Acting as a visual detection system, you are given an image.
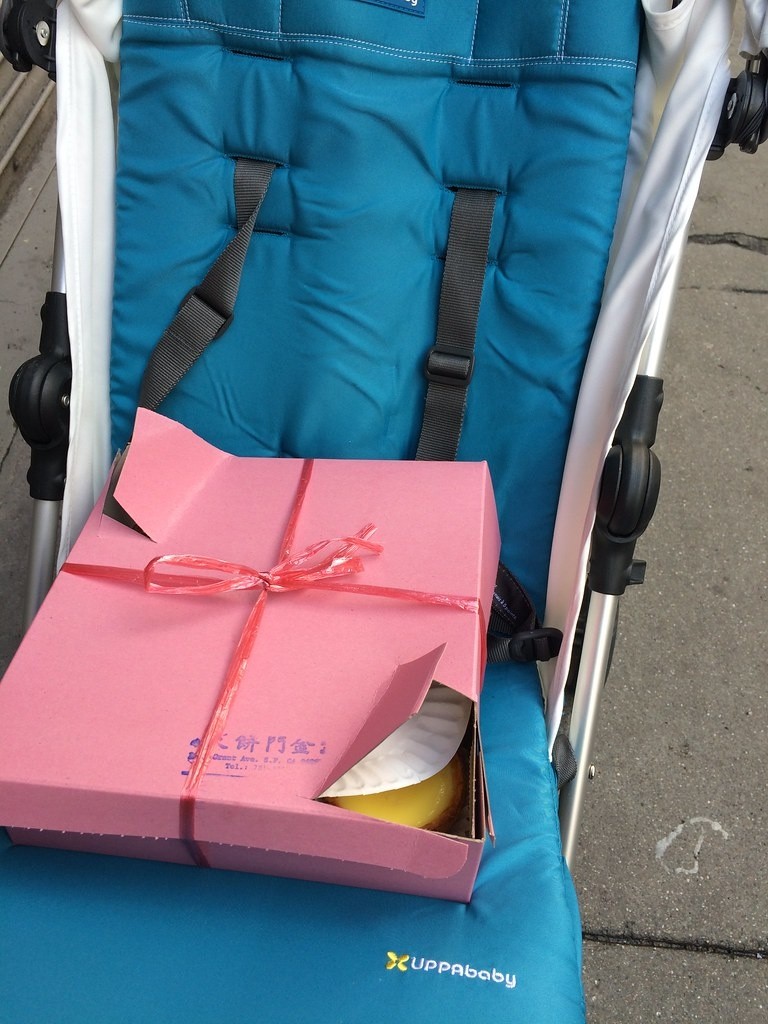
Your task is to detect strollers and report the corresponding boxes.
[1,0,765,1022]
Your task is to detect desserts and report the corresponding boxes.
[330,750,461,832]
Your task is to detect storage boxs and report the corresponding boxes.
[1,407,503,904]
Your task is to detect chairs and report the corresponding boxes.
[0,0,768,1024]
